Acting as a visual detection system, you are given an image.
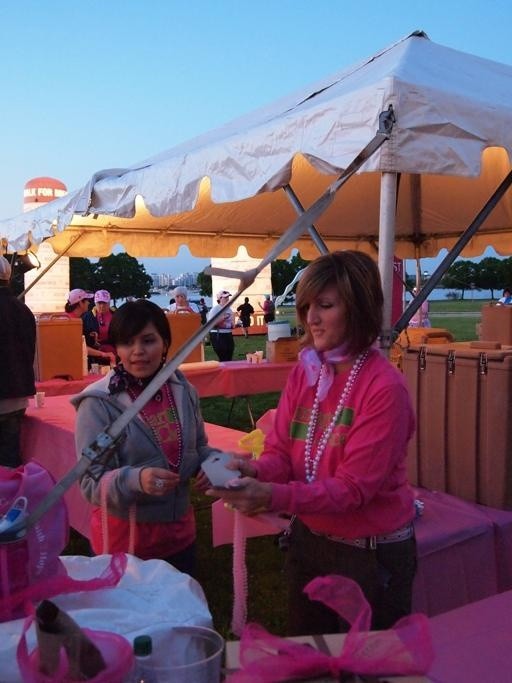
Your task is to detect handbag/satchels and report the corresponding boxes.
[0,458,70,599]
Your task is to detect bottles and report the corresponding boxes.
[237,318,243,327]
[126,634,158,682]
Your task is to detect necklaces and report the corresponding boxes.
[129,381,183,468]
[304,347,370,482]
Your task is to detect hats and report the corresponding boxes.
[94,289,111,302]
[264,294,270,299]
[217,290,232,300]
[68,288,95,306]
[0,256,11,280]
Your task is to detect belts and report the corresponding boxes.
[311,521,414,550]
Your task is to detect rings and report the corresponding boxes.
[239,459,243,471]
[155,479,164,488]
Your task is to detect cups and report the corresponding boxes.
[33,391,45,408]
[89,362,111,375]
[246,349,264,364]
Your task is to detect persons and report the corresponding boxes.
[496,288,512,306]
[0,255,36,470]
[69,299,224,579]
[65,286,275,373]
[206,251,418,636]
[407,287,431,326]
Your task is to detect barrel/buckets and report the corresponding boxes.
[268,321,291,340]
[0,536,33,621]
